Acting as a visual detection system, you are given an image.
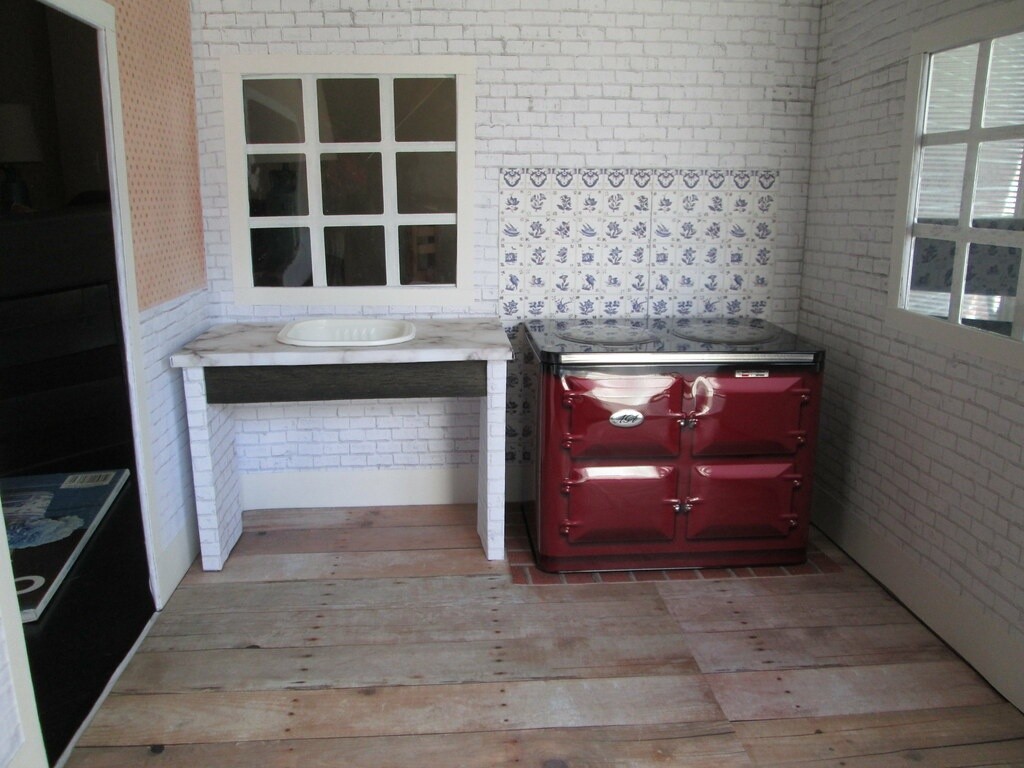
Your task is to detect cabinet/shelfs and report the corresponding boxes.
[531,362,822,574]
